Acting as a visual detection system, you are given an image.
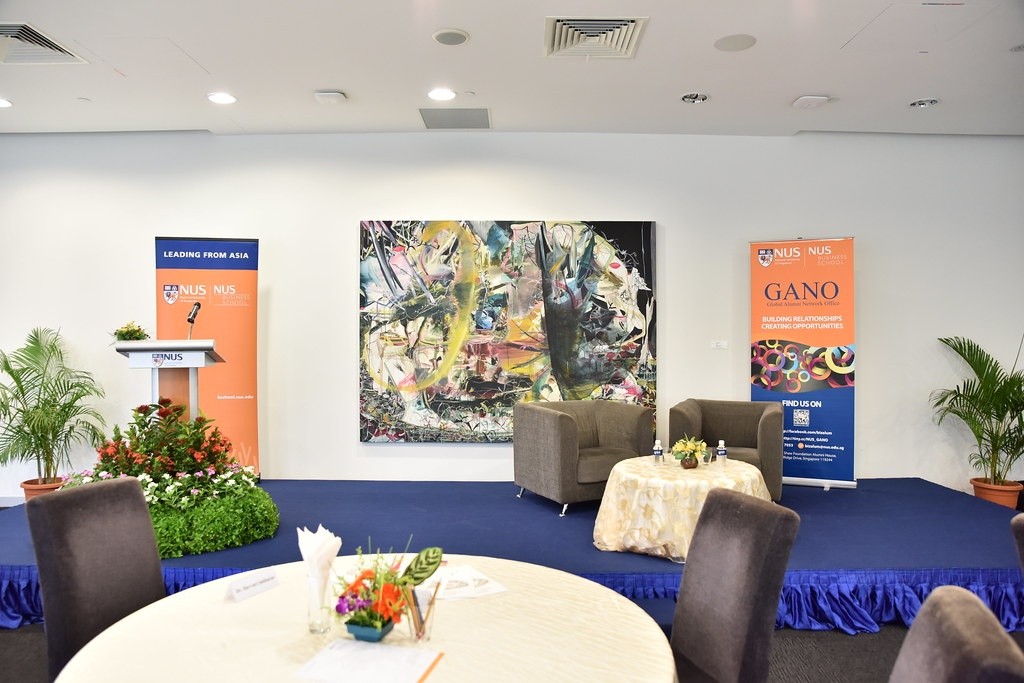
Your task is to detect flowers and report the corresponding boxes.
[671,433,709,458]
[54,399,282,557]
[331,537,448,628]
[114,321,145,335]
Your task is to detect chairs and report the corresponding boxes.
[28,479,166,683]
[886,586,1024,683]
[668,489,801,683]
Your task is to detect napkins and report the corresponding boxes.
[297,525,343,608]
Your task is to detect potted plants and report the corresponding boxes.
[0,327,111,502]
[926,336,1024,509]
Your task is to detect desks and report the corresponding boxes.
[594,456,775,563]
[56,553,681,683]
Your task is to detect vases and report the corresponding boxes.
[121,331,142,341]
[348,616,392,641]
[680,454,699,469]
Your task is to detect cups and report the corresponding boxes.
[662,448,672,462]
[305,598,332,633]
[405,599,434,641]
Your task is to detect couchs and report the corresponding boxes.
[669,397,784,501]
[513,399,653,517]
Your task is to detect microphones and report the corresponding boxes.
[187,302,201,323]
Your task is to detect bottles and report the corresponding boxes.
[653,440,663,466]
[716,440,727,468]
